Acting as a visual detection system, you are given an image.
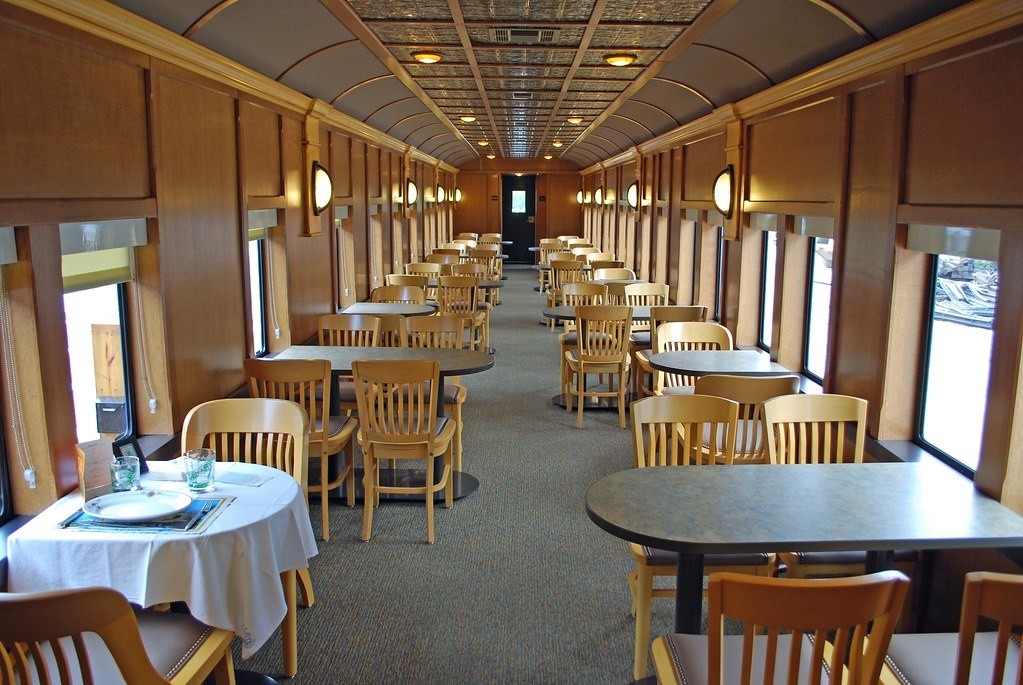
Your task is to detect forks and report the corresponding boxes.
[185,500,213,531]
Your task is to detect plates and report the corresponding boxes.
[83,490,192,522]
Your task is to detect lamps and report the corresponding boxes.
[410,51,445,64]
[627,180,638,211]
[312,160,332,215]
[487,153,496,159]
[544,153,552,160]
[455,187,462,202]
[476,140,489,146]
[407,177,418,207]
[565,116,585,123]
[594,186,603,207]
[553,141,563,147]
[602,53,638,67]
[437,182,445,205]
[458,116,476,123]
[576,190,583,204]
[712,163,734,220]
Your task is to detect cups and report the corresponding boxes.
[109,456,142,493]
[183,448,215,494]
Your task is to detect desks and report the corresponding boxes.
[341,303,435,346]
[542,304,660,409]
[528,246,571,291]
[6,459,319,677]
[421,278,504,354]
[459,253,509,305]
[530,264,591,324]
[649,349,792,419]
[585,461,1023,685]
[272,345,495,502]
[477,240,513,280]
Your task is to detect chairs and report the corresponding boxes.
[0,229,1022,685]
